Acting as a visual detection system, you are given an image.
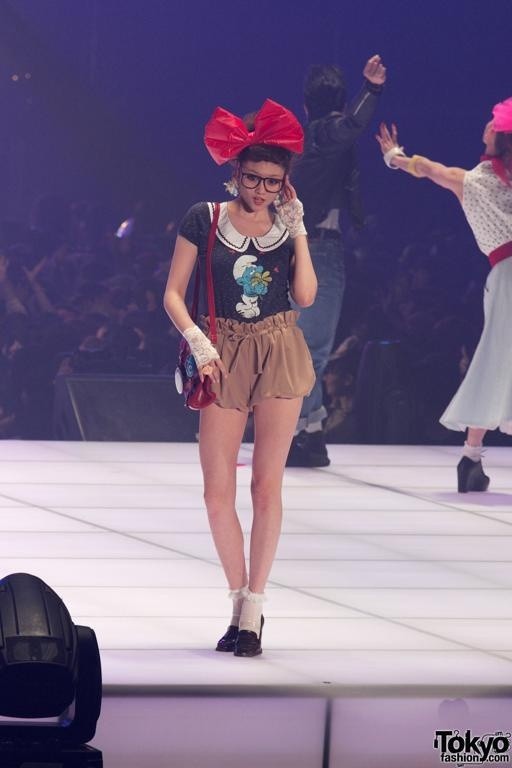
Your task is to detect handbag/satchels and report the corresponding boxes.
[174,336,217,411]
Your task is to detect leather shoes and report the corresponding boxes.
[216,624,239,653]
[234,614,265,657]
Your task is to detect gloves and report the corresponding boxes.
[274,197,309,239]
[182,324,221,368]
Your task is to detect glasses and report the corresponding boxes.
[236,160,285,194]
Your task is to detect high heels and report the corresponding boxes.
[457,455,490,494]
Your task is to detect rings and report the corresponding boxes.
[202,364,213,375]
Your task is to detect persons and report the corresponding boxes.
[162,96,321,657]
[374,93,512,494]
[0,188,493,443]
[284,53,389,468]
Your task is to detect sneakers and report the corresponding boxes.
[284,427,329,468]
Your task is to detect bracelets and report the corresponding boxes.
[383,145,406,170]
